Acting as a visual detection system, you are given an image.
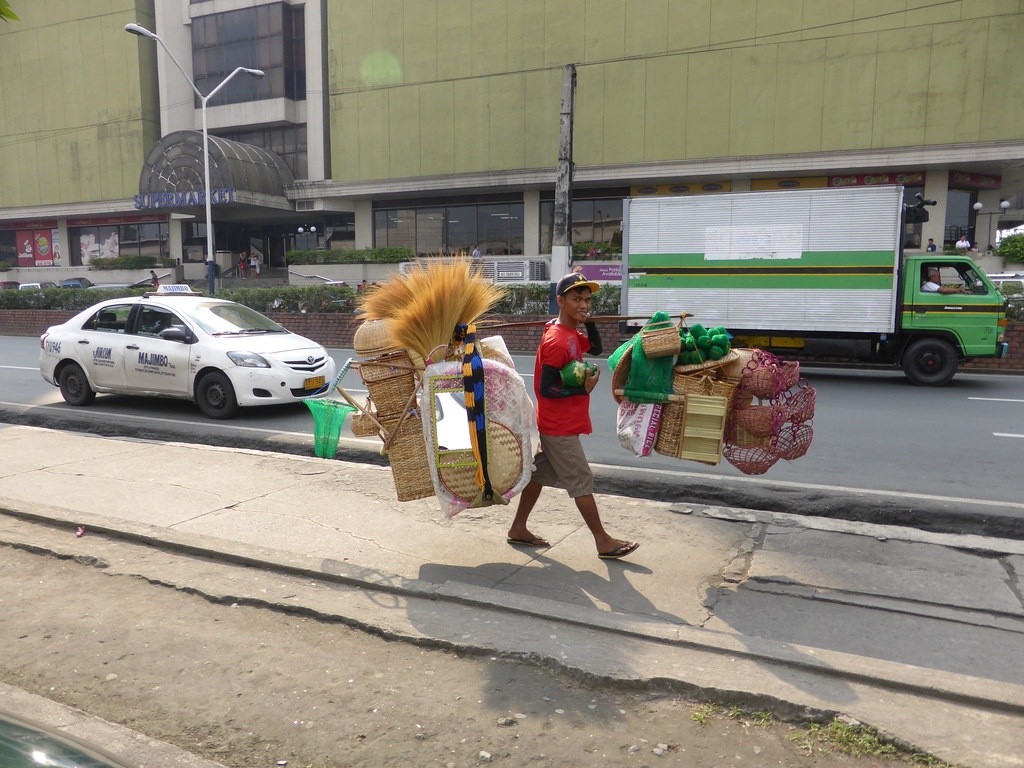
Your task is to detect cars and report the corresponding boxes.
[38,283,336,422]
[61,277,95,290]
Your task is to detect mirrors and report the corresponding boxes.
[429,375,489,468]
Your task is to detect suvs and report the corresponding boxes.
[975,273,1024,311]
[18,281,61,290]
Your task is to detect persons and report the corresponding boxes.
[150,270,159,286]
[926,238,936,252]
[240,250,257,279]
[921,269,964,293]
[505,273,641,558]
[967,242,980,252]
[473,245,480,257]
[956,234,970,248]
[359,279,367,291]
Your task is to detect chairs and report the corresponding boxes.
[97,312,123,328]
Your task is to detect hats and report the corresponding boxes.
[928,269,940,276]
[556,272,600,296]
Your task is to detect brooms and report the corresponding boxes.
[345,247,511,367]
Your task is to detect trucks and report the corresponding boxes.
[616,184,1010,389]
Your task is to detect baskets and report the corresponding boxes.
[351,398,380,437]
[722,348,818,474]
[359,351,436,502]
[652,371,736,466]
[641,321,680,358]
[354,318,405,351]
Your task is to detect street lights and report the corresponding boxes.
[972,201,1011,253]
[123,23,266,299]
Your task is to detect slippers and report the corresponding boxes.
[598,541,639,559]
[507,535,550,547]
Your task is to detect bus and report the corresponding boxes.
[0,282,22,291]
